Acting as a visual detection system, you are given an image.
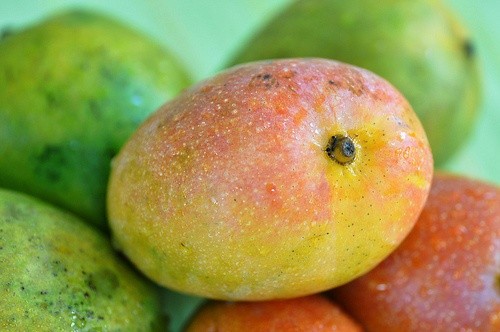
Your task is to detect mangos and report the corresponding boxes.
[0,1,500,332]
